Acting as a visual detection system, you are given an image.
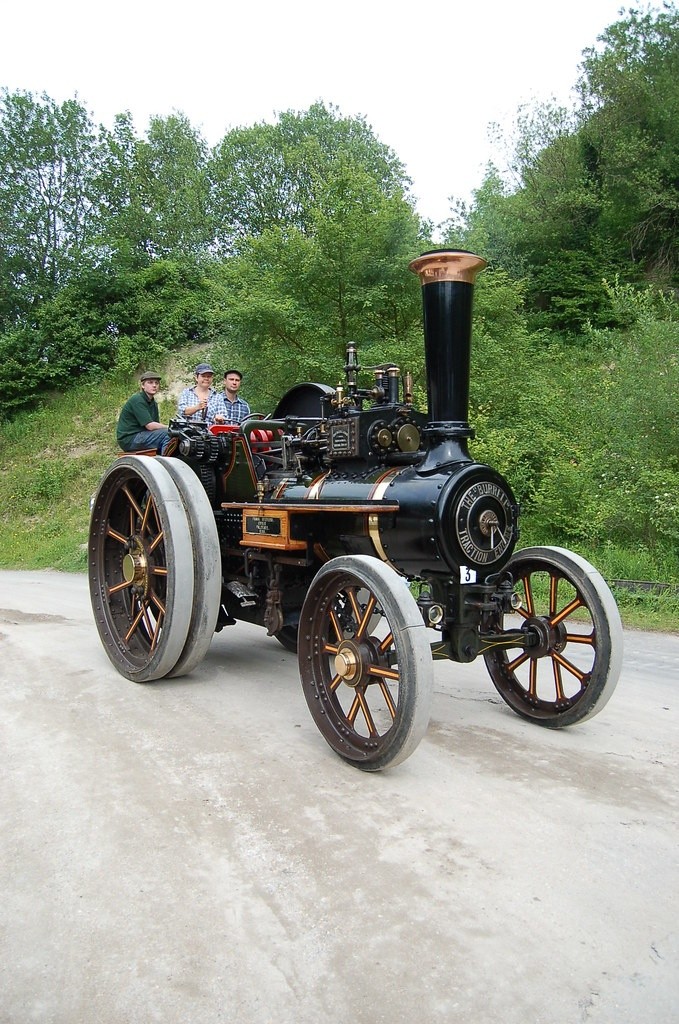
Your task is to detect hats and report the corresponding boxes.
[140,370,162,381]
[195,363,215,374]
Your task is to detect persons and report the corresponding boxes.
[220,369,251,426]
[116,372,172,456]
[177,363,228,428]
